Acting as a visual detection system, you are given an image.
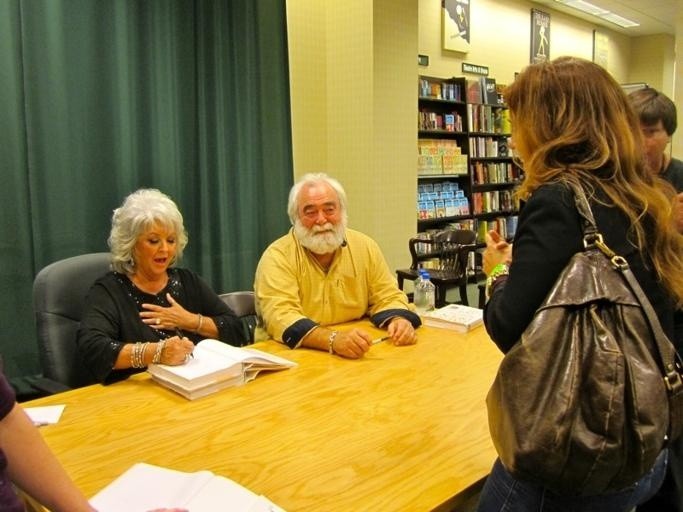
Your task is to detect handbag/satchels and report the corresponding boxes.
[485,235,679,505]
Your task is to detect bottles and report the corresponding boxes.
[411,268,435,312]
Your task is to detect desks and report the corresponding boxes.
[9,301,521,511]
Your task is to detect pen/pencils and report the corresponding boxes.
[372,336,391,344]
[176,329,194,359]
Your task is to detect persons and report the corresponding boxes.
[476,58,683,512]
[628,87,683,233]
[0,363,189,512]
[75,189,246,386]
[253,173,422,360]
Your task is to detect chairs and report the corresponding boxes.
[217,288,267,346]
[29,248,124,396]
[395,226,491,312]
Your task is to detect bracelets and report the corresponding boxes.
[131,339,165,370]
[328,328,337,355]
[486,264,510,287]
[195,315,202,333]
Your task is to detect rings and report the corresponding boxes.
[186,354,190,361]
[156,318,161,324]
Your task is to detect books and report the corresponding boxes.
[417,77,520,276]
[147,338,298,388]
[151,363,289,401]
[422,304,484,333]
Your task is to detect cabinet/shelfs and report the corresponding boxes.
[463,76,524,249]
[415,69,480,292]
